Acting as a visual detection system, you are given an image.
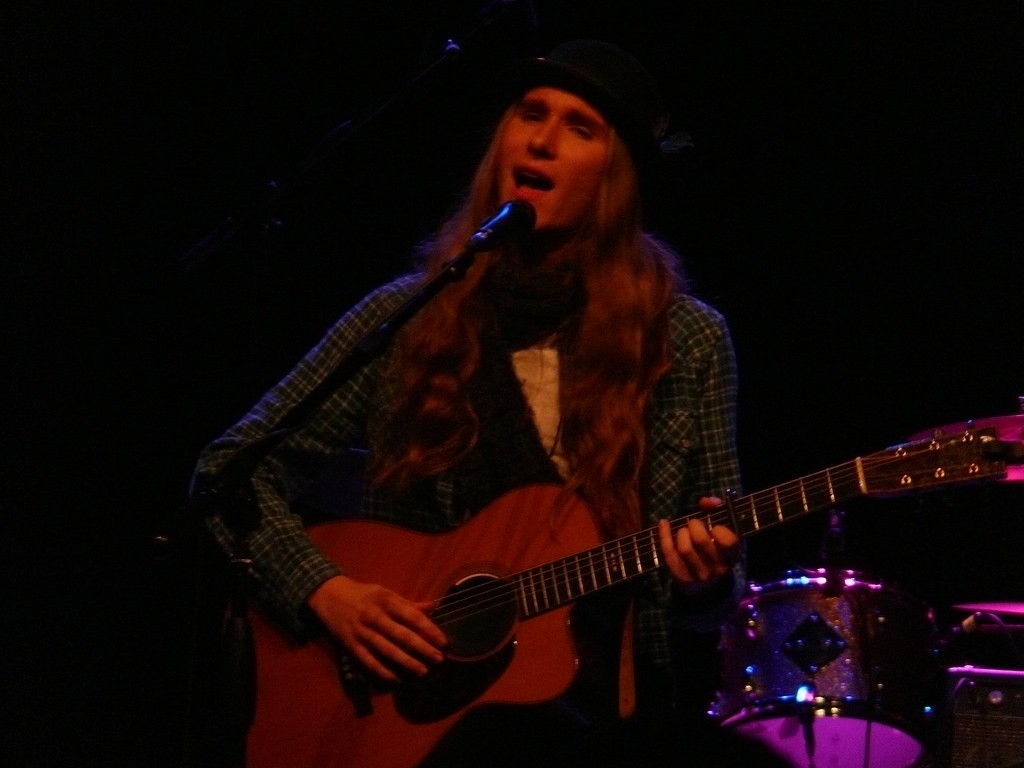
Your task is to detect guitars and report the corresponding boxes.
[247,418,1007,768]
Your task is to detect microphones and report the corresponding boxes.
[469,199,537,252]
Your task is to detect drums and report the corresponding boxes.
[706,565,943,768]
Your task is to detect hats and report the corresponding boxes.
[509,35,669,180]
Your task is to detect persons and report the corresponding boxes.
[187,53,798,768]
